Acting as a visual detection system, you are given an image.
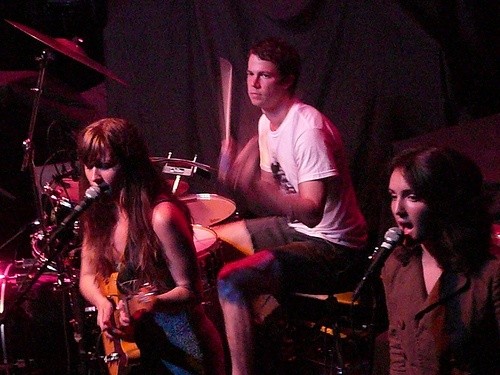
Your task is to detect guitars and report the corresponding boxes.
[97,271,157,375]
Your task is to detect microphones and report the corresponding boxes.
[351,227,405,300]
[54,187,104,236]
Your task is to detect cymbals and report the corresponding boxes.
[2,18,130,90]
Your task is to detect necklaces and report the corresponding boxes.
[119,211,131,222]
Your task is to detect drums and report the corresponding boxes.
[176,194,238,227]
[167,179,189,194]
[191,224,222,302]
[0,258,82,356]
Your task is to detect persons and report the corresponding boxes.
[372,143,500,375]
[205,40,371,375]
[74,117,222,375]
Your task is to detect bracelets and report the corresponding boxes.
[287,196,296,221]
